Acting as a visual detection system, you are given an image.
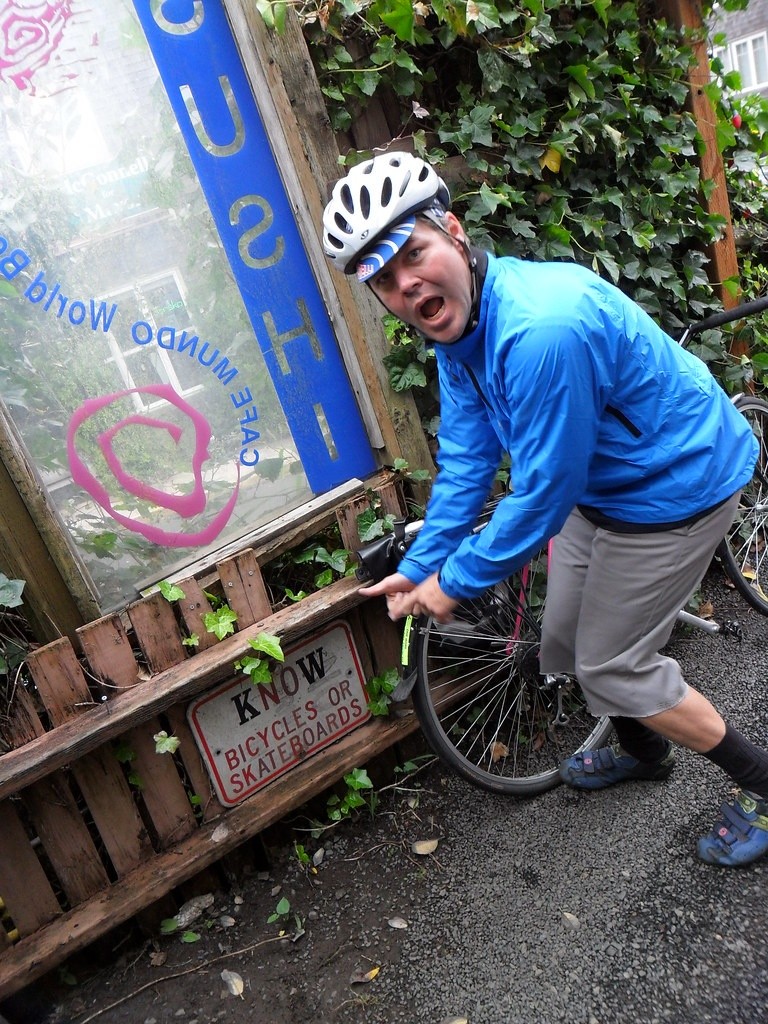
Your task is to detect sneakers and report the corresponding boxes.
[696,789,768,866]
[559,737,675,789]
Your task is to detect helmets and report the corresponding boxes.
[323,151,450,274]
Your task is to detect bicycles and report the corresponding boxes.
[354,299,767,799]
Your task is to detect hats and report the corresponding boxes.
[345,196,445,284]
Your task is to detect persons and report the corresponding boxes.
[320,152,768,868]
